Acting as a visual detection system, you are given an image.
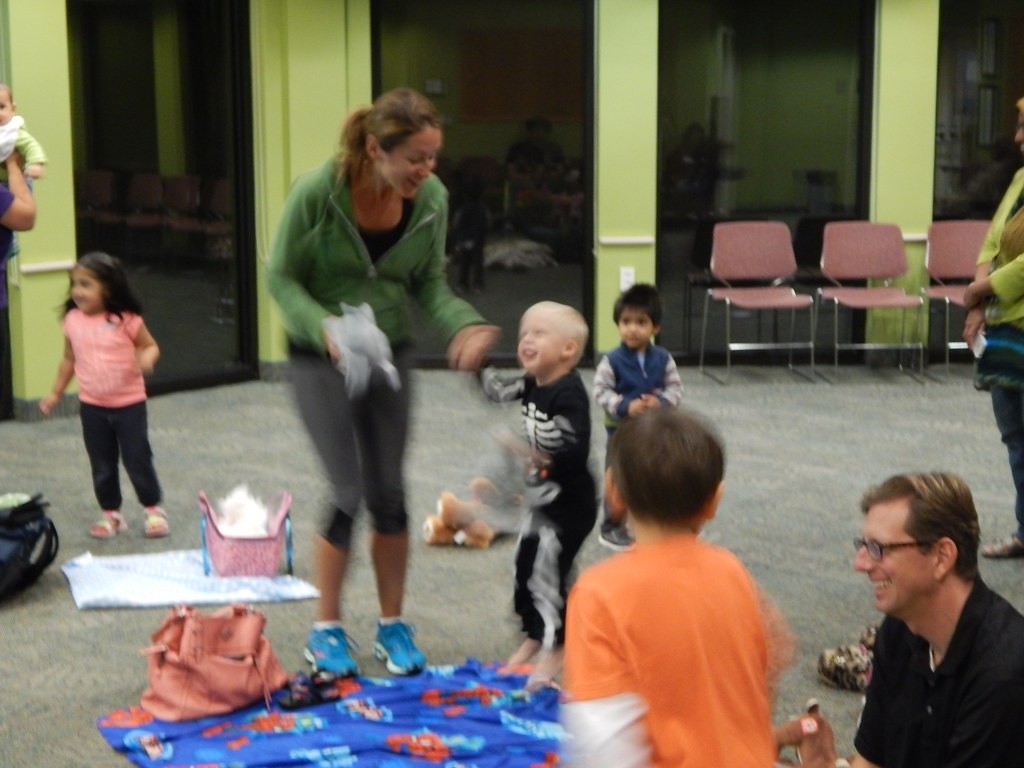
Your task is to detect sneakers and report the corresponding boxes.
[303,627,364,677]
[371,619,426,676]
[598,520,638,552]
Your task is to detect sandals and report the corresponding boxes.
[142,506,169,538]
[89,510,130,539]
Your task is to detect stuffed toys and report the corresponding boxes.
[423,475,526,549]
[817,628,878,696]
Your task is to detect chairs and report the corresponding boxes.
[698,221,819,385]
[677,217,780,354]
[812,222,927,386]
[910,220,992,387]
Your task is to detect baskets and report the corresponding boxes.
[197,488,293,578]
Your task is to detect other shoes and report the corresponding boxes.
[982,535,1024,559]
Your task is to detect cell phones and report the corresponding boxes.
[971,330,987,358]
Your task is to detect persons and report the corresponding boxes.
[842,471,1023,768]
[464,301,599,682]
[263,88,497,678]
[963,101,1024,558]
[36,252,171,539]
[548,406,797,766]
[1,82,46,259]
[591,284,686,552]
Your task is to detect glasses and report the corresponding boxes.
[853,536,932,561]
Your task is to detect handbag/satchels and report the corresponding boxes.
[139,603,289,722]
[0,493,60,603]
